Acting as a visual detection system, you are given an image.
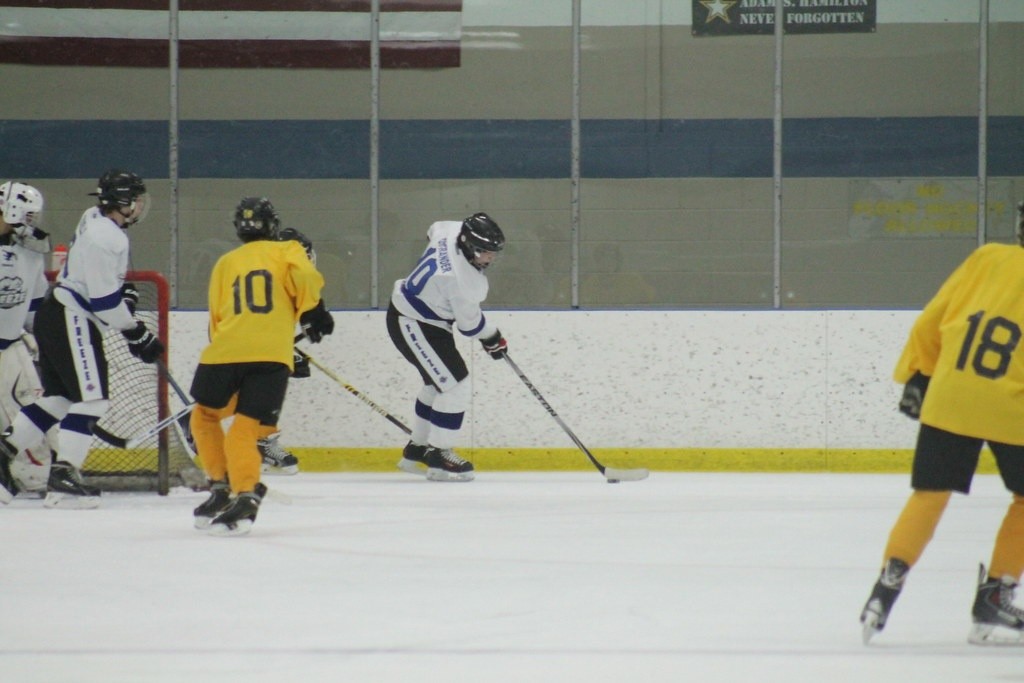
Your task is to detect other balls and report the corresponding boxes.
[607,478,620,483]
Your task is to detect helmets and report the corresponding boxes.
[457,212,506,272]
[279,227,316,269]
[0,181,43,228]
[1016,201,1024,247]
[87,169,152,230]
[233,195,281,244]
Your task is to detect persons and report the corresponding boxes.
[0,168,334,537]
[857,201,1024,647]
[386,211,509,483]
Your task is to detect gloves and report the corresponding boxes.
[120,319,165,364]
[122,282,140,317]
[898,370,931,419]
[290,347,311,379]
[299,298,336,345]
[478,327,508,359]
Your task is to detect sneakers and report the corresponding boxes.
[0,436,102,510]
[193,479,268,537]
[256,429,299,476]
[967,562,1024,645]
[860,558,910,644]
[174,408,203,470]
[396,440,474,481]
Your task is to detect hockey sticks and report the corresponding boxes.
[90,325,316,452]
[155,361,190,404]
[293,347,412,435]
[500,350,650,481]
